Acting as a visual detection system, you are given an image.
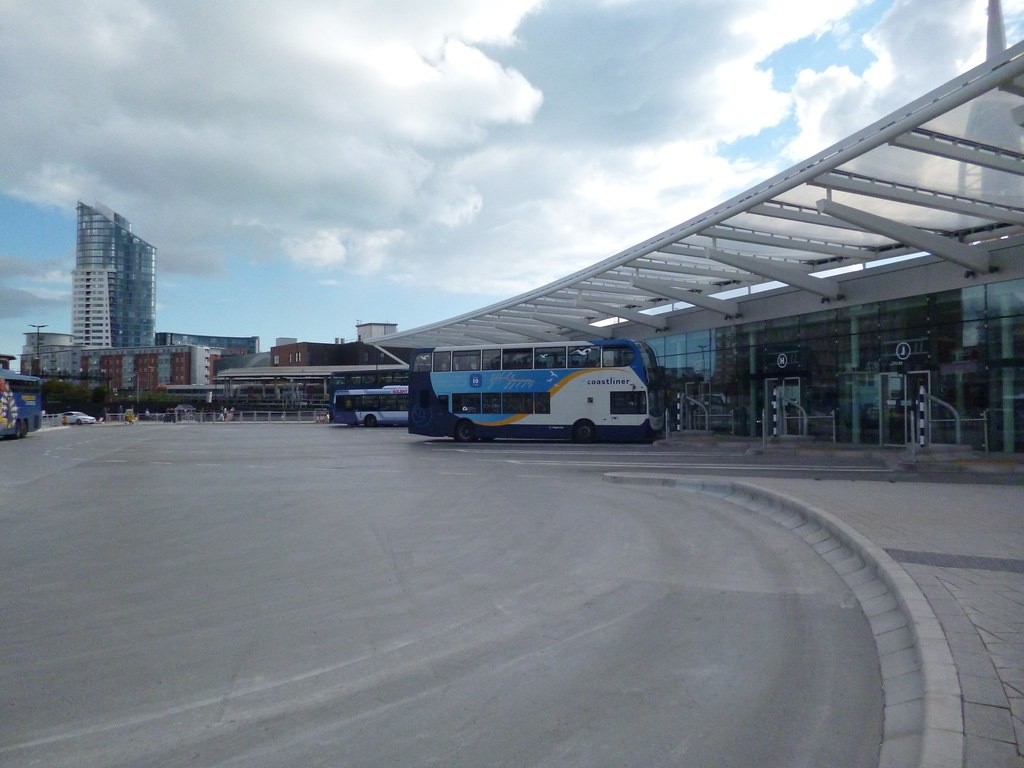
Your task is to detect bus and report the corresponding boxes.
[327,369,408,423]
[333,385,409,428]
[407,339,666,445]
[0,368,42,439]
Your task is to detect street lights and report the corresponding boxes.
[29,325,47,358]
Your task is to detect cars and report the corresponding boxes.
[174,404,196,412]
[62,411,96,425]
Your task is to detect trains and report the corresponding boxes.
[155,383,330,409]
